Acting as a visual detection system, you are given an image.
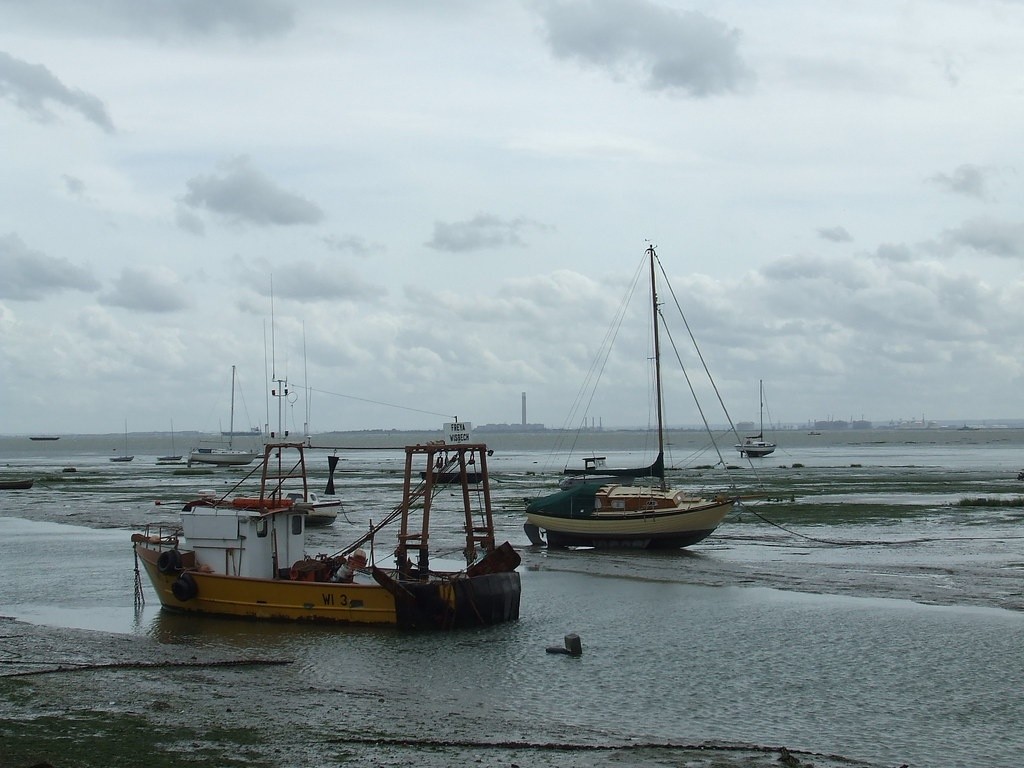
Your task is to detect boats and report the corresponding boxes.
[0,480,33,488]
[133,379,520,625]
[815,414,1007,429]
[29,436,60,440]
[250,490,341,524]
[806,431,821,435]
[63,467,76,472]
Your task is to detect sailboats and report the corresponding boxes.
[188,365,258,470]
[523,245,741,550]
[157,417,183,460]
[734,379,778,457]
[109,419,134,462]
